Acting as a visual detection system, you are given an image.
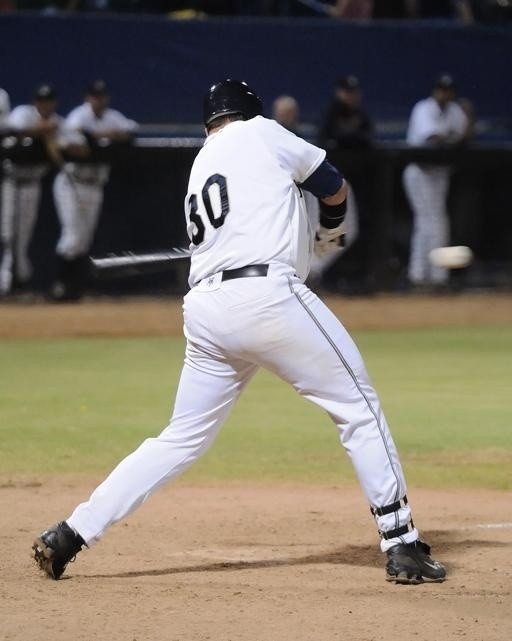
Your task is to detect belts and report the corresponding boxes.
[195,264,269,285]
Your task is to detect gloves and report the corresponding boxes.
[316,224,344,259]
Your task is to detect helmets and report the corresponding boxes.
[203,77,263,128]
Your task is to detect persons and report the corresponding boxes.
[29,75,448,585]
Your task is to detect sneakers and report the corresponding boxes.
[29,520,82,580]
[386,539,445,582]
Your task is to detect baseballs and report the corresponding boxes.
[430,245,474,268]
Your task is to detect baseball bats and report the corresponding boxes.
[90,233,347,270]
